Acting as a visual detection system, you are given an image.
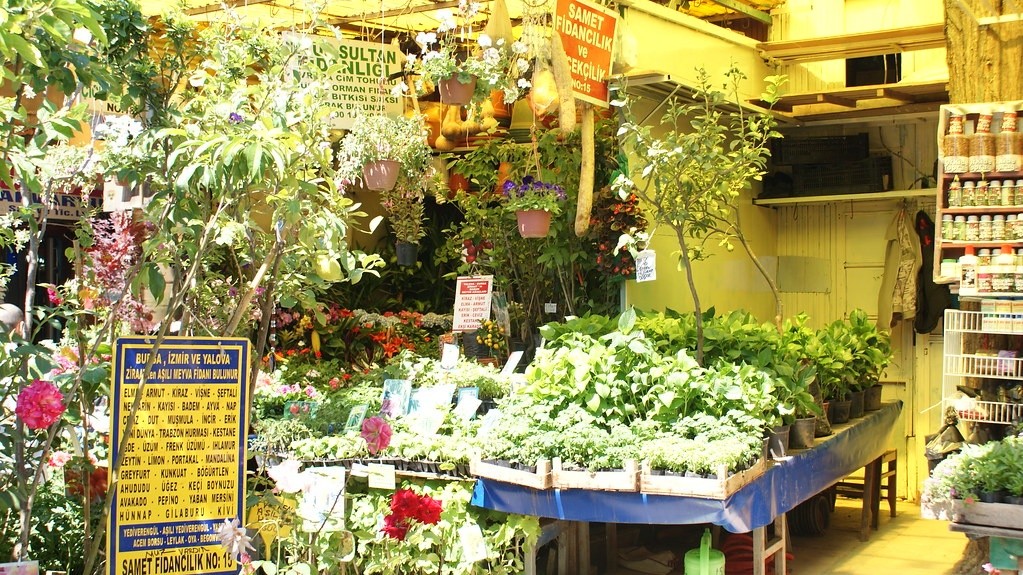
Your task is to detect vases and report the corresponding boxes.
[514,209,552,238]
[979,433,1023,505]
[362,157,402,192]
[436,72,479,106]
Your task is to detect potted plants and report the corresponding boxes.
[468,304,893,501]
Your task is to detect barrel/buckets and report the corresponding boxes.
[683,528,725,575]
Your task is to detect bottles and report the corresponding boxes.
[995,245,1014,265]
[960,244,977,296]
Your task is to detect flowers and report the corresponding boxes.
[500,174,565,214]
[335,112,438,246]
[2,0,707,575]
[924,447,978,506]
[388,0,533,107]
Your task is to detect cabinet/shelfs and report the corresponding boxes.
[932,101,1023,431]
[583,0,948,132]
